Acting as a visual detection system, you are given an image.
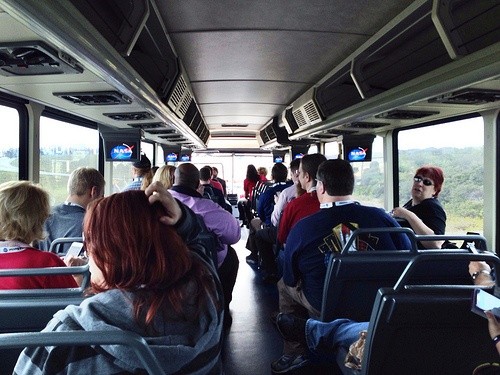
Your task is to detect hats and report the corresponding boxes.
[132,154,151,169]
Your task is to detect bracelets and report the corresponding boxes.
[493,335,500,345]
[472,269,490,280]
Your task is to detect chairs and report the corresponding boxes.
[252,180,500,375]
[0,236,168,375]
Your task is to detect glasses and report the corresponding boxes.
[296,170,304,174]
[315,179,321,184]
[414,175,434,187]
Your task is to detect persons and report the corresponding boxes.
[121,155,241,310]
[0,180,85,290]
[236,154,500,375]
[36,168,106,254]
[12,182,224,375]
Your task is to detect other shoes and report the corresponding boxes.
[222,313,232,328]
[246,254,258,260]
[241,222,247,227]
[253,272,270,282]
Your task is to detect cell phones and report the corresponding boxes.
[470,286,500,319]
[64,242,84,267]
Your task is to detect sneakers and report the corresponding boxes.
[270,351,309,373]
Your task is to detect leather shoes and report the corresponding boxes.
[273,312,307,343]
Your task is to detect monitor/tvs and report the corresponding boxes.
[344,141,372,162]
[164,149,179,162]
[273,155,284,162]
[292,151,307,159]
[180,153,191,161]
[104,140,138,162]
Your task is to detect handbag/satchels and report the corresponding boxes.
[344,332,367,370]
